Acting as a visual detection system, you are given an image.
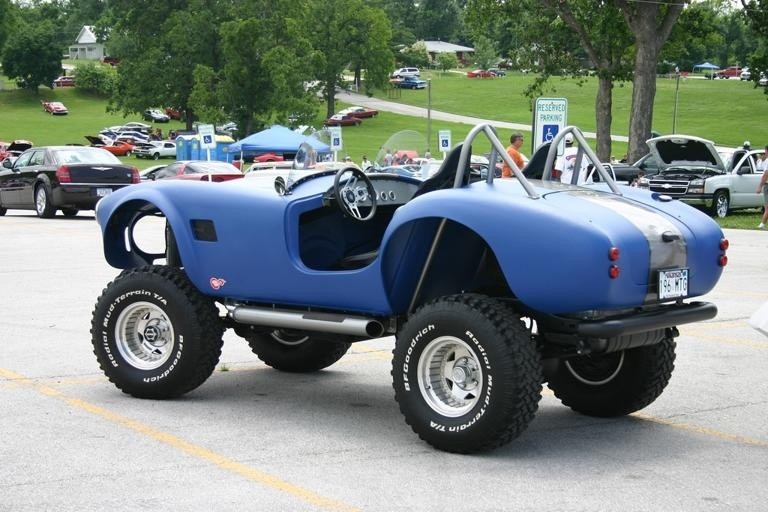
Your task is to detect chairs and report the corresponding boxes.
[510,141,556,181]
[339,145,472,270]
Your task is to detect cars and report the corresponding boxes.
[467,68,505,78]
[54,75,75,87]
[42,101,69,115]
[323,107,378,127]
[741,66,768,86]
[143,106,186,123]
[0,141,245,218]
[16,78,39,88]
[389,66,427,89]
[85,122,196,160]
[216,119,239,135]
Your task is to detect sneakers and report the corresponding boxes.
[757,222,765,229]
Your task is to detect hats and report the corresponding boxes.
[564,132,573,143]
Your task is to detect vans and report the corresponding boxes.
[100,56,118,66]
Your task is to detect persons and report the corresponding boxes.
[621,154,627,163]
[342,145,431,172]
[631,171,651,191]
[554,133,589,186]
[755,153,767,170]
[755,144,767,228]
[146,127,177,142]
[740,140,757,173]
[501,131,525,178]
[610,155,618,163]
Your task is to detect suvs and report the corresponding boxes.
[718,66,742,79]
